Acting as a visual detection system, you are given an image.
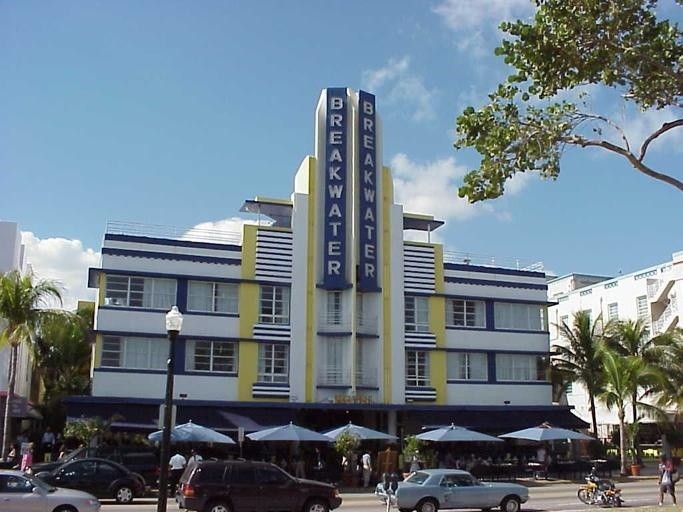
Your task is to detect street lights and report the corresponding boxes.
[154,303,184,511]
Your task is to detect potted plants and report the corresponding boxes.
[332,425,361,488]
[621,419,641,476]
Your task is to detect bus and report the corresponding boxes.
[606,424,681,459]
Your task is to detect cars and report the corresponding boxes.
[0,468,101,511]
[374,467,529,511]
[31,457,147,504]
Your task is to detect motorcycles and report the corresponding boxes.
[576,460,624,507]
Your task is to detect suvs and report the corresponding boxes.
[174,457,341,511]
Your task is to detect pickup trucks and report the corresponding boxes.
[23,442,158,486]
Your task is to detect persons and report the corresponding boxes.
[442,449,489,470]
[657,454,676,507]
[536,443,548,464]
[360,448,372,489]
[6,425,61,462]
[168,449,187,498]
[187,447,203,467]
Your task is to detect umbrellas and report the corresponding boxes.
[403,422,506,454]
[148,419,237,456]
[496,420,596,480]
[245,421,332,472]
[321,420,401,443]
